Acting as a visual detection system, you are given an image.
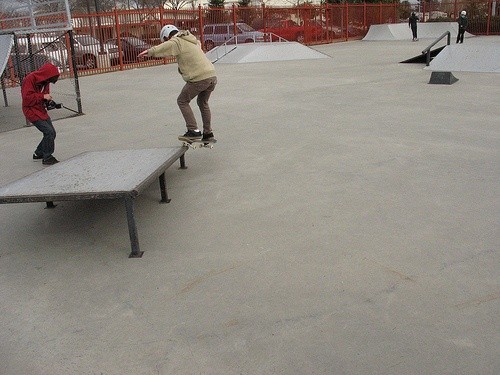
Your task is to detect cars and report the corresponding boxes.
[103,36,158,64]
[309,18,345,40]
[347,20,368,34]
[55,34,126,70]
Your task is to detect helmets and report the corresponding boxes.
[160,25,179,43]
[461,11,466,15]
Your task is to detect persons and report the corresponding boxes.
[137,25,217,142]
[21,62,59,165]
[456,10,468,44]
[409,12,419,41]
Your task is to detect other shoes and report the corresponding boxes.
[33,153,44,162]
[179,130,202,138]
[42,155,60,167]
[202,132,215,146]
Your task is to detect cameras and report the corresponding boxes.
[44,99,63,110]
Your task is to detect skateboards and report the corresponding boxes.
[178,137,218,150]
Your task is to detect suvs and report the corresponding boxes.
[257,17,326,43]
[9,35,69,74]
[200,22,272,51]
